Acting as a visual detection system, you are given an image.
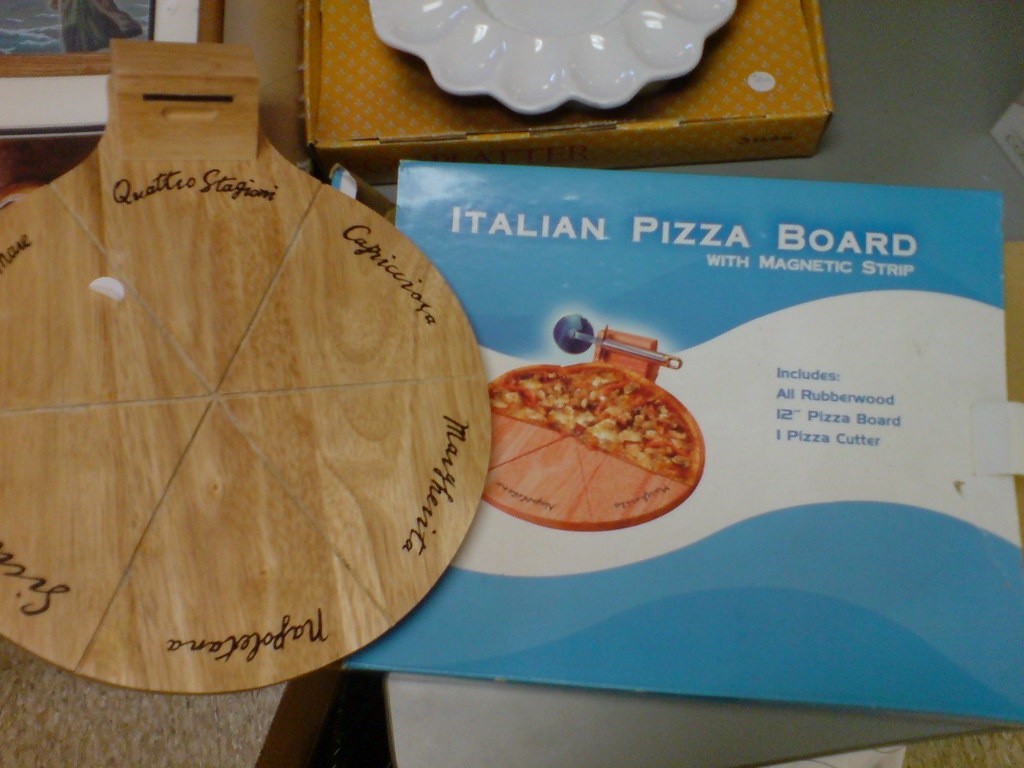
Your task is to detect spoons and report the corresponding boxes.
[554,313,683,371]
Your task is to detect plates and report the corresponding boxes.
[370,1,738,116]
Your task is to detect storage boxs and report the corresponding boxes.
[305,0,835,188]
[328,157,1023,724]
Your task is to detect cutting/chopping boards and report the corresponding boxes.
[481,327,706,532]
[1,38,492,695]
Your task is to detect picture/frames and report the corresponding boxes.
[0,1,225,138]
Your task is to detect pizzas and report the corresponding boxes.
[487,365,702,488]
